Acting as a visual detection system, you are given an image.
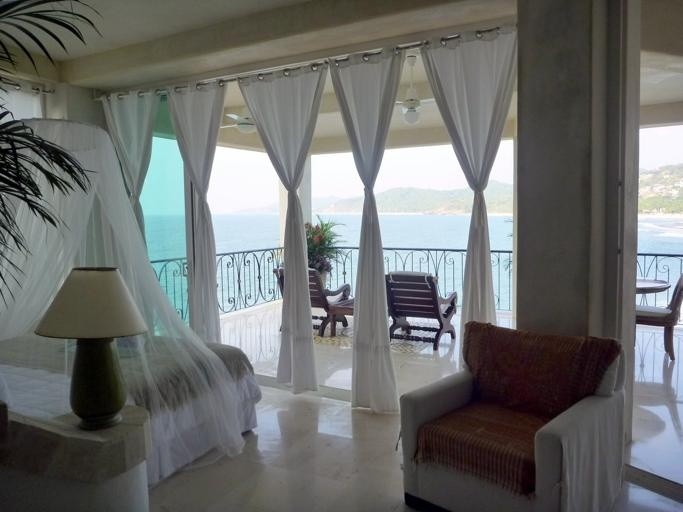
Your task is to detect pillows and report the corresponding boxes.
[464,322,621,413]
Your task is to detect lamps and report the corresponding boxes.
[34,267,148,428]
[405,109,419,124]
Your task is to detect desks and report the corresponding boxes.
[635,280,669,293]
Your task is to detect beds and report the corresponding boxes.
[0,335,262,489]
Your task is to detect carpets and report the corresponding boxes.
[312,321,434,355]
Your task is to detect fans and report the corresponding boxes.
[396,56,435,109]
[220,113,258,135]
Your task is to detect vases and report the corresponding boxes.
[315,271,326,287]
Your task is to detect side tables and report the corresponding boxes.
[327,300,354,336]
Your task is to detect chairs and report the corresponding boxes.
[273,268,349,337]
[636,275,682,361]
[385,273,457,349]
[401,321,625,512]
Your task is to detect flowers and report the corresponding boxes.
[304,213,348,273]
